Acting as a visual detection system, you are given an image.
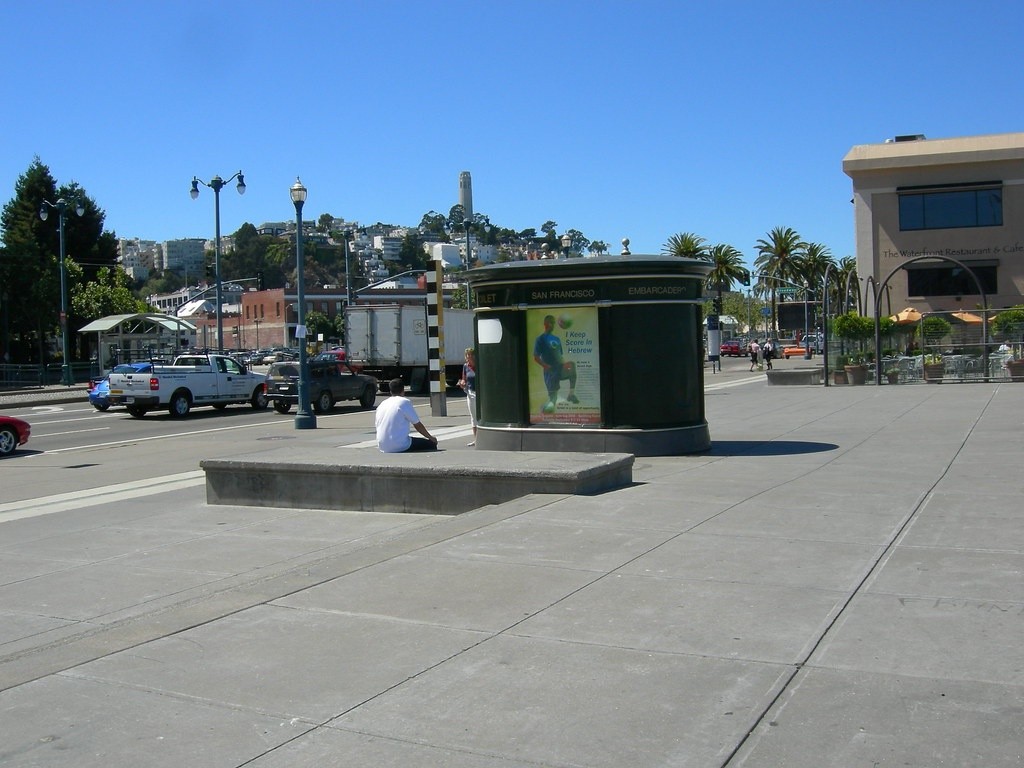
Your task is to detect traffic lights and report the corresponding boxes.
[743,272,750,286]
[712,296,723,313]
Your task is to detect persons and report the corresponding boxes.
[748,339,761,372]
[906,342,920,356]
[999,341,1010,367]
[460,349,476,446]
[764,339,773,370]
[375,379,438,452]
[534,317,579,413]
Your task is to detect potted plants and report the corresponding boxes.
[988,304,1024,382]
[833,349,848,385]
[885,363,900,383]
[917,316,951,384]
[833,310,869,385]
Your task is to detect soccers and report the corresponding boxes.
[540,402,555,415]
[558,313,574,330]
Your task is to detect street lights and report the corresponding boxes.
[803,280,811,360]
[188,170,246,354]
[562,235,572,259]
[289,175,317,429]
[39,195,85,386]
[449,217,491,311]
[251,320,263,350]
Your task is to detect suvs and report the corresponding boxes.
[263,362,379,415]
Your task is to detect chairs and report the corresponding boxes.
[192,359,203,365]
[865,354,1010,384]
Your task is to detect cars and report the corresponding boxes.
[758,340,782,359]
[146,346,363,374]
[720,340,748,357]
[0,415,31,457]
[794,331,824,354]
[89,362,161,412]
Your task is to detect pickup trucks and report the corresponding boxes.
[105,355,269,420]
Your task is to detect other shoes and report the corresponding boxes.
[567,395,580,404]
[468,441,475,446]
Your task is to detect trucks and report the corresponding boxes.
[345,303,477,394]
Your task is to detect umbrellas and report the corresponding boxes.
[887,307,924,329]
[988,314,996,323]
[952,308,982,346]
[918,309,968,353]
